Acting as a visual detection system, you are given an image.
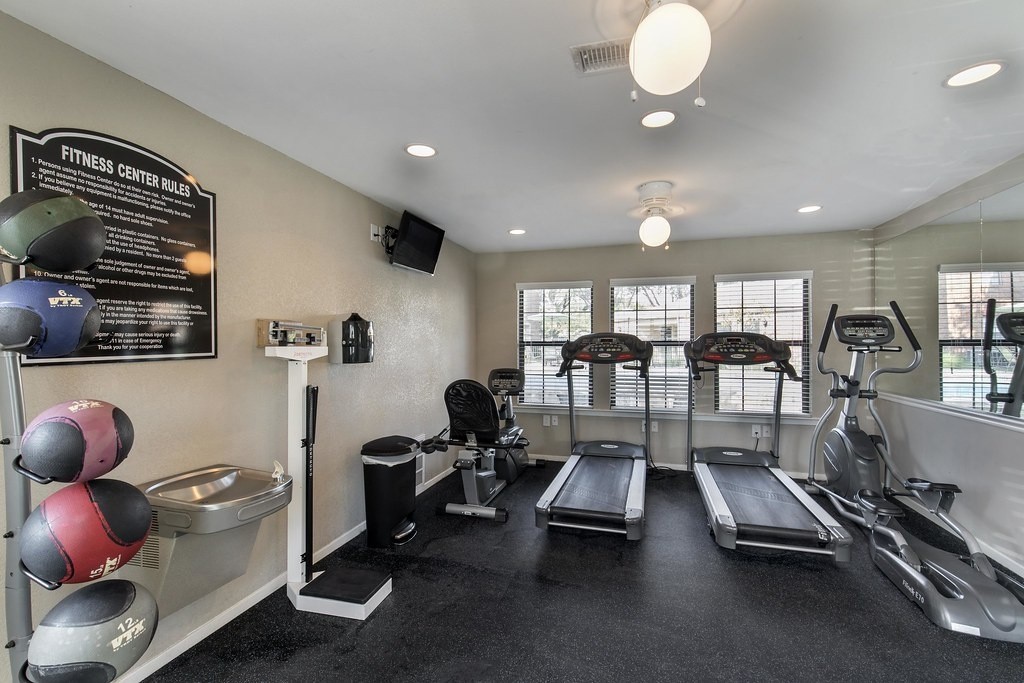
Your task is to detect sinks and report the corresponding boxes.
[114,463,294,621]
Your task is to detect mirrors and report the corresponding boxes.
[875,181,1024,422]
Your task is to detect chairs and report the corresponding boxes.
[445,379,510,443]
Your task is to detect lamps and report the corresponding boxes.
[628,1,711,108]
[638,182,672,251]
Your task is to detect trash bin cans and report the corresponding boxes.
[360,435,422,547]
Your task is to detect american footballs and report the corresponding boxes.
[19,478,153,583]
[0,189,107,273]
[0,276,102,356]
[20,398,134,482]
[27,578,160,683]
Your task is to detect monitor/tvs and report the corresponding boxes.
[390,210,445,276]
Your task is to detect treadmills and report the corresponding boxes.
[534,332,653,540]
[683,331,855,565]
